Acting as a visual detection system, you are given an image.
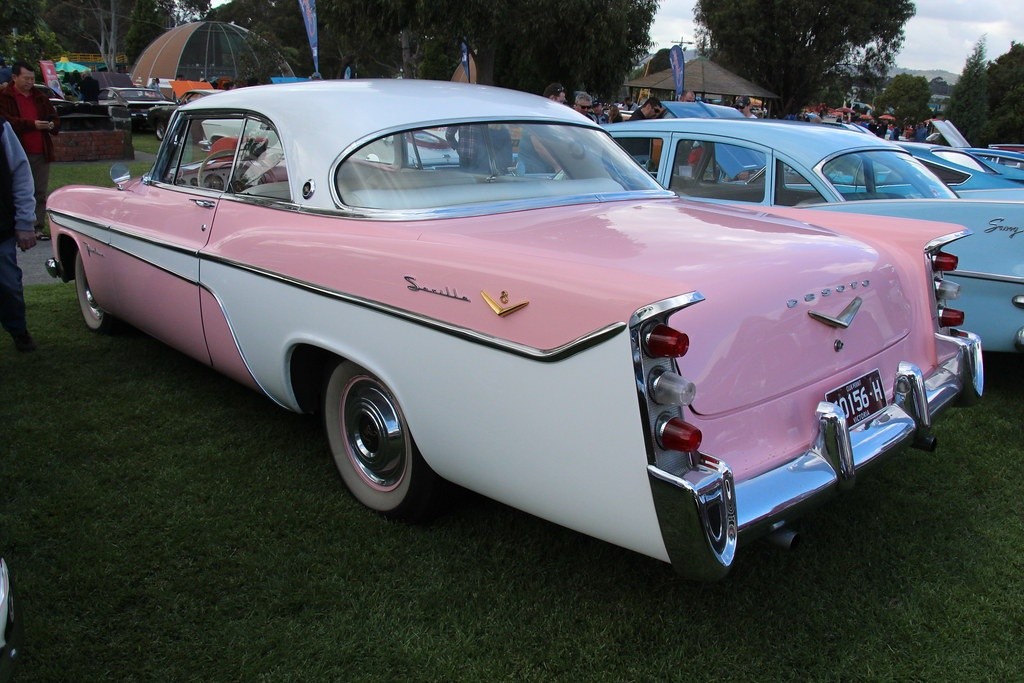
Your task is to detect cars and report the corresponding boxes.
[511,101,1024,352]
[33,71,463,170]
[44,79,984,576]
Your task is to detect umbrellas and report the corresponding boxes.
[834,108,855,113]
[879,115,895,119]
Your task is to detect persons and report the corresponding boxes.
[628,97,662,164]
[869,120,888,139]
[623,96,639,111]
[687,141,702,177]
[889,123,899,141]
[0,114,37,352]
[224,82,236,90]
[445,120,512,173]
[516,83,566,174]
[915,122,927,142]
[0,56,60,241]
[573,92,623,125]
[148,77,160,90]
[733,95,757,118]
[836,116,842,122]
[62,70,100,102]
[680,90,695,102]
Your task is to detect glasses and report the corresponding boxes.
[552,87,565,95]
[653,107,660,114]
[578,104,593,111]
[735,106,745,109]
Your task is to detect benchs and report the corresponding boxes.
[336,161,615,210]
[685,180,790,205]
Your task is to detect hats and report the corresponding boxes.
[733,95,750,106]
[592,100,602,106]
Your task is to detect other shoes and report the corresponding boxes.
[10,326,37,352]
[34,224,52,241]
